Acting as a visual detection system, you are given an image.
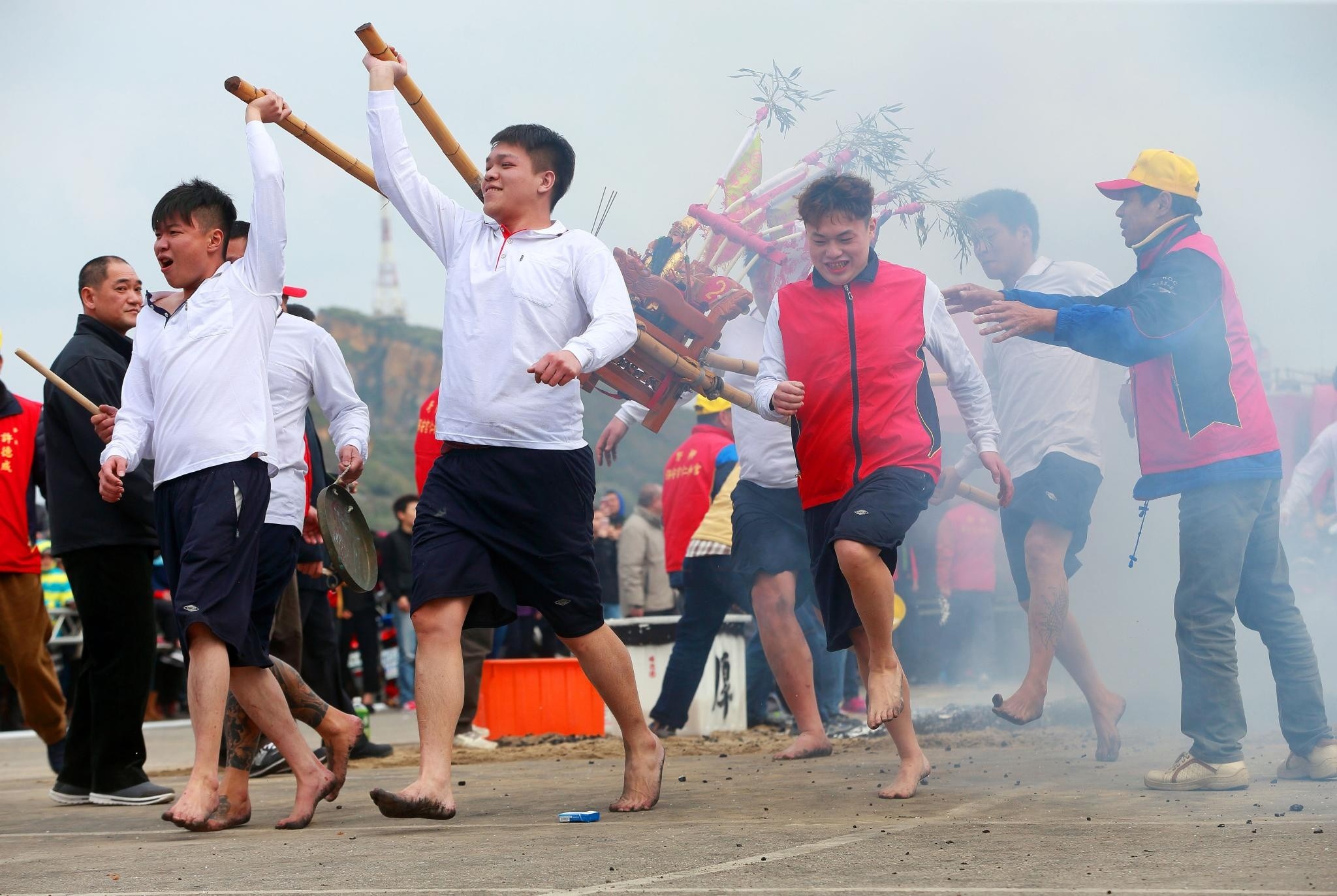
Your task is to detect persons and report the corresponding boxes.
[927,189,1130,761]
[936,147,1337,789]
[746,171,1014,799]
[362,43,666,820]
[0,89,572,833]
[590,383,1019,738]
[1258,360,1337,732]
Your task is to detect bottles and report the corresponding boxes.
[352,697,370,741]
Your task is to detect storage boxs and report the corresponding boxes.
[561,609,750,740]
[474,659,608,741]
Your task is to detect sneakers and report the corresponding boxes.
[89,778,175,806]
[1276,737,1337,779]
[50,780,92,805]
[247,743,287,779]
[1144,752,1249,792]
[315,745,328,763]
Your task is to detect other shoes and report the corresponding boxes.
[453,724,498,750]
[347,733,392,759]
[48,702,74,773]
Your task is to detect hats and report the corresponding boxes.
[694,391,732,416]
[1094,149,1202,203]
